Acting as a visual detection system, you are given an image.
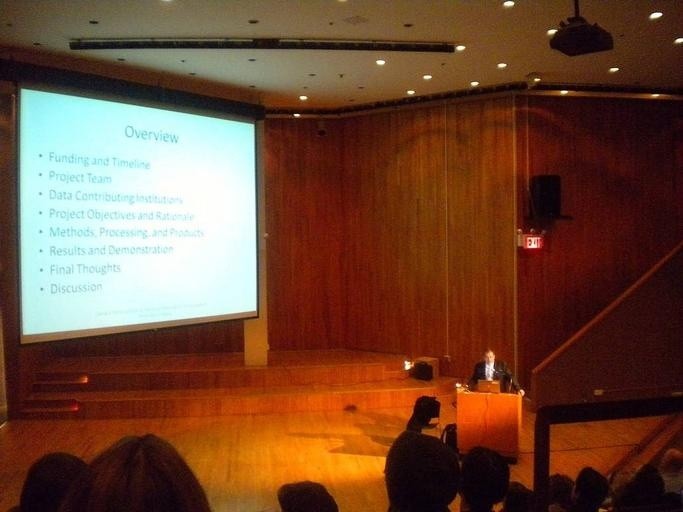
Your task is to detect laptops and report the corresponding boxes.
[478,380,500,393]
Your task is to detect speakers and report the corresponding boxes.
[531,176,562,217]
[415,364,432,380]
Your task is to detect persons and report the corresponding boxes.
[466,348,525,396]
[21,432,210,511]
[277,480,339,512]
[385,398,683,512]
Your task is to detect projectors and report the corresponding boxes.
[550,24,613,57]
